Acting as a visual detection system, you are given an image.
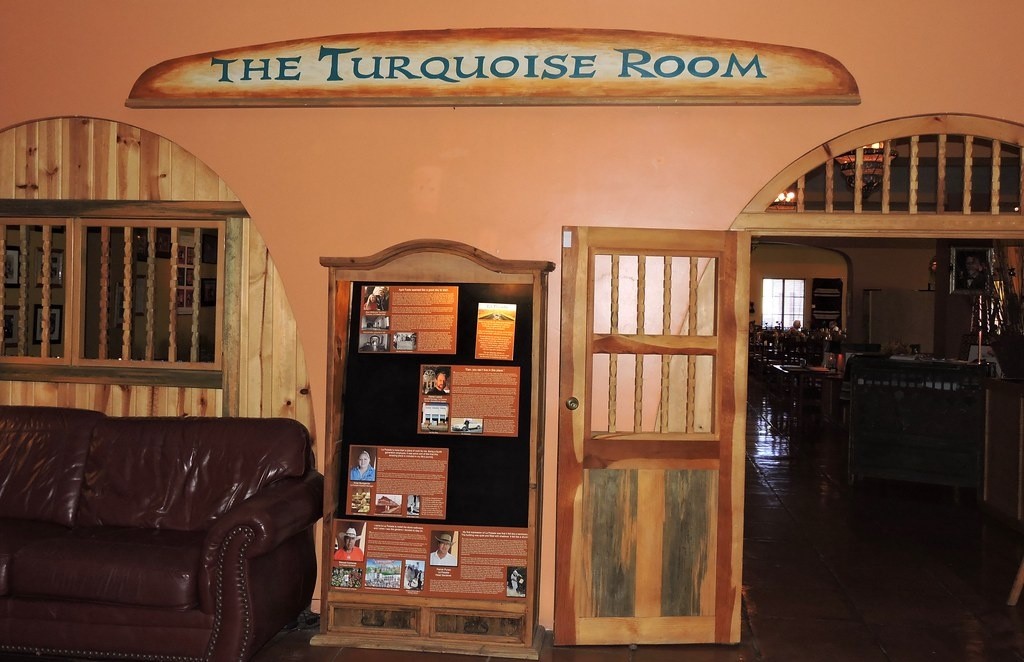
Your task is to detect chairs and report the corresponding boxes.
[749,325,892,434]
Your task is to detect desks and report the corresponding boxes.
[983,379,1024,537]
[772,363,844,427]
[889,353,966,365]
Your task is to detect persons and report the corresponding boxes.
[410,500,414,513]
[430,534,458,566]
[787,320,841,337]
[385,505,390,511]
[511,570,523,592]
[350,448,377,482]
[333,527,363,561]
[342,571,350,587]
[361,495,367,506]
[364,290,416,351]
[405,562,423,590]
[427,367,448,395]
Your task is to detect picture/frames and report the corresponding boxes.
[3,305,20,348]
[32,303,63,345]
[33,245,64,289]
[112,233,217,329]
[948,240,996,296]
[4,245,23,289]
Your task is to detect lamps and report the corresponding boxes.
[931,256,937,273]
[832,138,899,199]
[769,181,808,211]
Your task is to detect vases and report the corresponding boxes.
[989,333,1024,381]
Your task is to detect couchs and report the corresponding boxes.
[0,404,324,662]
